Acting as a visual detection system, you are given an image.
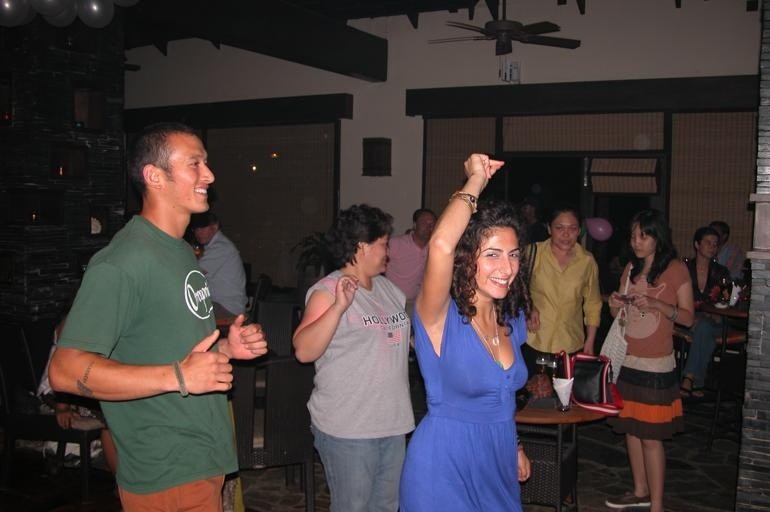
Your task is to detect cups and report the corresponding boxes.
[555,386,572,412]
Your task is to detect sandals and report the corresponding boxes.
[692,388,705,398]
[679,377,694,397]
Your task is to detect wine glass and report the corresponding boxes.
[535,353,549,376]
[549,358,561,380]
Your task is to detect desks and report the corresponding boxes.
[698,300,748,450]
[512,405,608,511]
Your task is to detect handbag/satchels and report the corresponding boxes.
[599,308,628,385]
[555,357,611,404]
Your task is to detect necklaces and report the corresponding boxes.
[471,304,501,363]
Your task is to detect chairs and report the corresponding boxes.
[0,322,105,509]
[673,326,748,416]
[215,274,317,511]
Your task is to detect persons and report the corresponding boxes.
[35,317,109,486]
[48,120,268,512]
[680,228,732,400]
[385,209,437,320]
[516,197,549,243]
[604,206,695,512]
[291,204,417,512]
[399,153,532,512]
[709,221,745,280]
[189,212,248,326]
[521,203,603,509]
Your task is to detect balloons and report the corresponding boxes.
[585,217,613,242]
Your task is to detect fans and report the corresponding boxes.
[428,0,580,49]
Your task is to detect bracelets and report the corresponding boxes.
[517,447,524,451]
[449,190,479,214]
[666,304,677,320]
[173,359,188,397]
[56,408,71,413]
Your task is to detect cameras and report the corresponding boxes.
[616,295,636,306]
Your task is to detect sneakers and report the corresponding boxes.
[605,491,651,509]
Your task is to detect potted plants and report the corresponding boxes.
[291,230,347,277]
